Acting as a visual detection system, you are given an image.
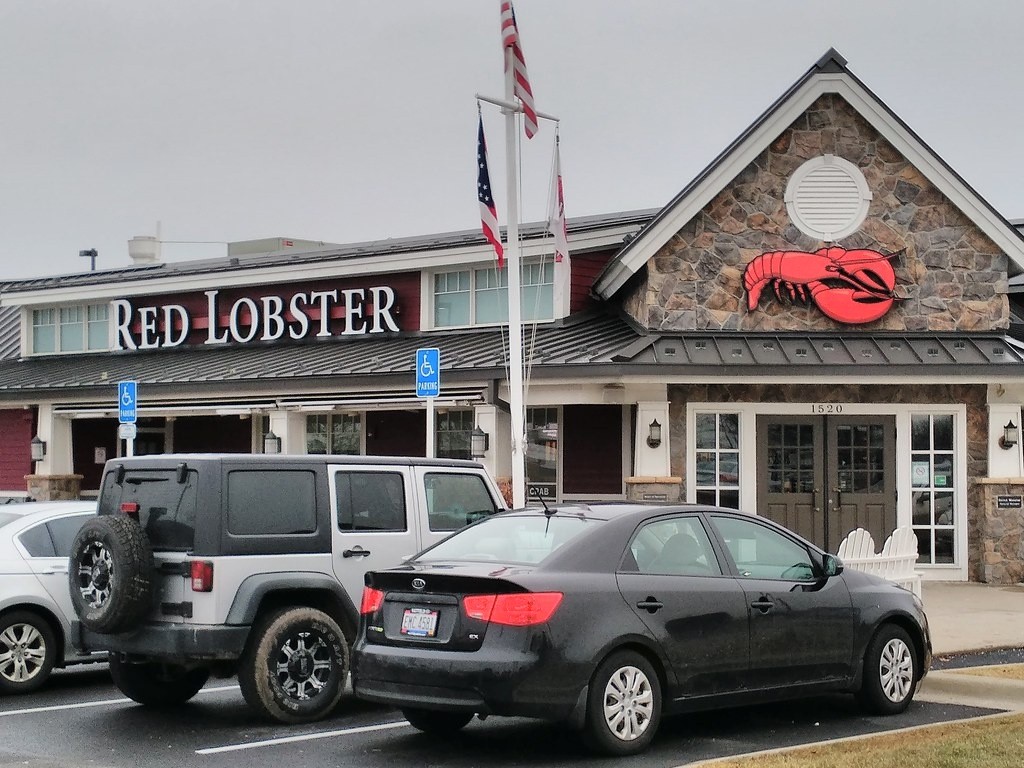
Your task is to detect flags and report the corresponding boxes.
[476,113,502,272]
[499,0,539,139]
[549,135,571,283]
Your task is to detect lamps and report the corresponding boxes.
[646,418,662,449]
[998,420,1018,451]
[470,424,489,459]
[264,429,281,455]
[29,435,46,462]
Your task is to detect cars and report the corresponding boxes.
[0,500,102,696]
[348,499,933,757]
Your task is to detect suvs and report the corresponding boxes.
[67,453,638,725]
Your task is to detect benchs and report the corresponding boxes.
[834,527,925,599]
